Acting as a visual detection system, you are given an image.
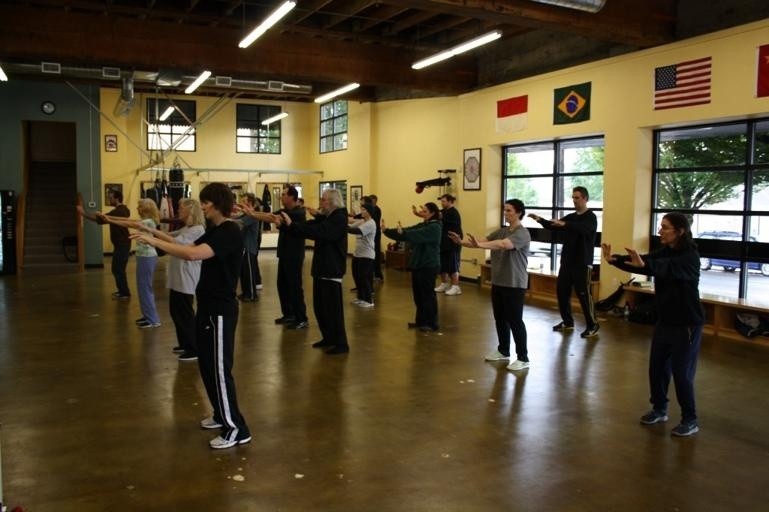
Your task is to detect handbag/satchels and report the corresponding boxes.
[737,311,769,337]
[628,294,657,325]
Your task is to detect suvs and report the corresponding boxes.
[696,232,769,276]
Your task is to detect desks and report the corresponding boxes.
[384,248,413,271]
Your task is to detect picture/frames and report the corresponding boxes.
[103,134,120,154]
[462,146,482,192]
[349,184,364,217]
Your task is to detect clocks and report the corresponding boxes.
[39,101,58,115]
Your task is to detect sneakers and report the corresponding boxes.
[485,351,510,360]
[640,410,668,425]
[582,327,598,338]
[670,422,698,436]
[239,279,463,354]
[508,360,529,371]
[112,292,252,448]
[554,323,576,331]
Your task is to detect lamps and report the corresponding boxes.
[406,5,506,73]
[231,0,298,53]
[153,90,177,120]
[181,41,210,94]
[261,97,288,126]
[307,28,364,104]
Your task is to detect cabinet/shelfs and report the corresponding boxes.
[476,259,600,312]
[621,279,768,349]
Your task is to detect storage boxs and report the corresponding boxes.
[63,235,78,262]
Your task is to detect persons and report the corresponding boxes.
[272,187,349,354]
[346,194,383,307]
[381,202,442,329]
[126,182,251,447]
[231,185,309,330]
[449,198,530,369]
[529,185,600,338]
[78,192,204,359]
[601,211,703,438]
[412,193,462,295]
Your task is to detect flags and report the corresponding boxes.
[494,94,529,134]
[651,56,716,111]
[753,44,769,97]
[550,83,593,126]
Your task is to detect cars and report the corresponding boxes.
[528,240,564,258]
